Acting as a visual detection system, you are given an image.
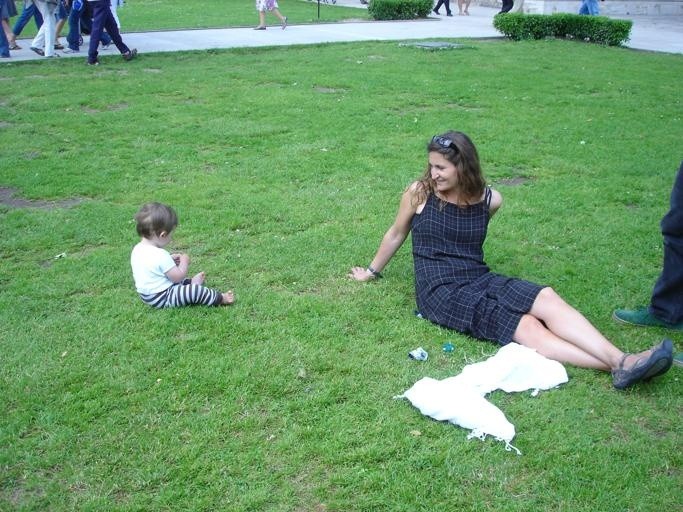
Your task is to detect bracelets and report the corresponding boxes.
[367,266,384,279]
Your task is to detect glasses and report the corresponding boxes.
[431,135,459,153]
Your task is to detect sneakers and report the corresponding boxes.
[31,43,78,59]
[282,17,288,30]
[87,62,100,66]
[433,9,452,16]
[612,308,683,330]
[102,42,110,49]
[7,34,20,50]
[122,49,137,61]
[673,353,683,364]
[612,339,673,388]
[253,26,266,30]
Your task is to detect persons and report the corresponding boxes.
[0,0,138,67]
[497,0,513,15]
[347,131,674,389]
[130,202,234,310]
[433,0,453,16]
[578,0,601,17]
[457,0,471,16]
[613,159,683,330]
[252,0,288,30]
